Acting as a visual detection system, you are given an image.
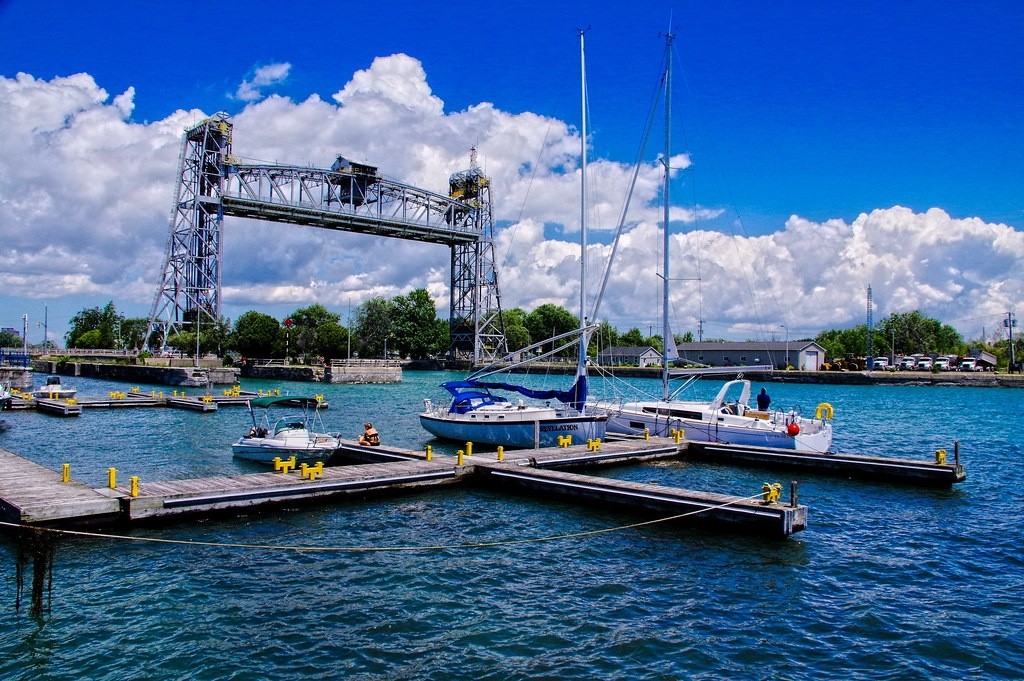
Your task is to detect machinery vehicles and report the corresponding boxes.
[821,351,866,371]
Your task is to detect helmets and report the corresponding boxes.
[366,423,371,426]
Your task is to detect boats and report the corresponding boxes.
[30,375,78,398]
[232,397,342,466]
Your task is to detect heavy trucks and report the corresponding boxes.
[874,353,996,372]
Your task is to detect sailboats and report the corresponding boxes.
[570,15,834,453]
[419,29,608,448]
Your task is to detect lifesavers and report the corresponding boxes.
[816,403,834,420]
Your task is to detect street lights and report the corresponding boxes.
[781,325,789,369]
[348,298,351,359]
[36,299,47,352]
[384,339,387,359]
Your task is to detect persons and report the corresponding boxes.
[357,421,382,447]
[755,387,771,412]
